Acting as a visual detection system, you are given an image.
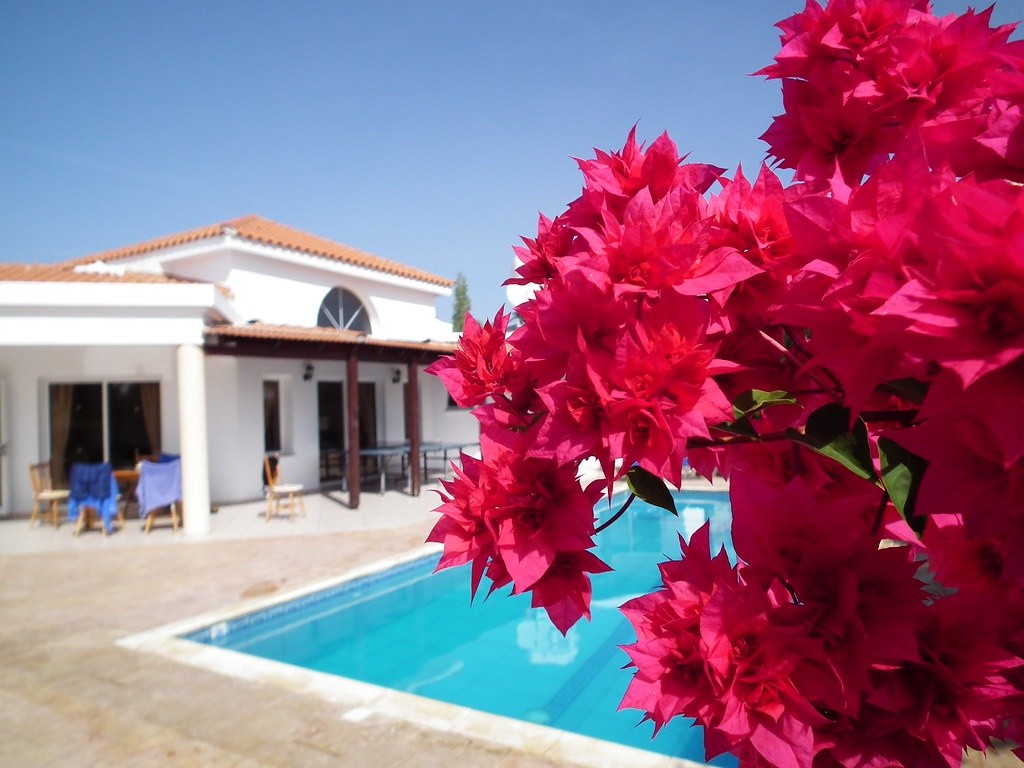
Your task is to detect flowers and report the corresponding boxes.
[423,0,1024,768]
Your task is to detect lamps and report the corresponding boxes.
[302,360,314,380]
[392,367,400,384]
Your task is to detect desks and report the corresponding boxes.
[343,443,484,496]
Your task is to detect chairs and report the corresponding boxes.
[260,453,306,531]
[26,460,70,530]
[67,462,124,539]
[134,457,180,537]
